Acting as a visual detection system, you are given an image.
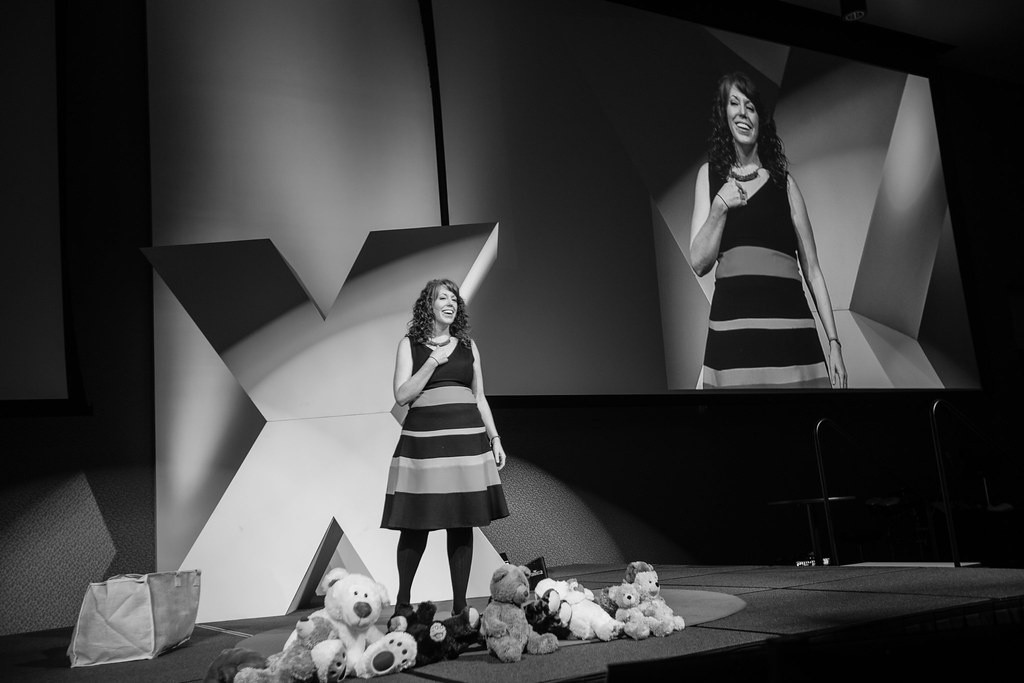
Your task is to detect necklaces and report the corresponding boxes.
[728,167,763,182]
[427,336,451,347]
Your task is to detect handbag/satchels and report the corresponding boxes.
[66,569,202,668]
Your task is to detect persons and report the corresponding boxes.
[380,278,510,620]
[691,70,849,388]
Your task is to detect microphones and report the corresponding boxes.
[434,309,444,314]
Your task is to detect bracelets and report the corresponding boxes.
[716,193,730,210]
[829,338,842,347]
[429,356,440,365]
[489,436,501,447]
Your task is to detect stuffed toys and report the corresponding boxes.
[203,568,488,683]
[480,561,685,663]
